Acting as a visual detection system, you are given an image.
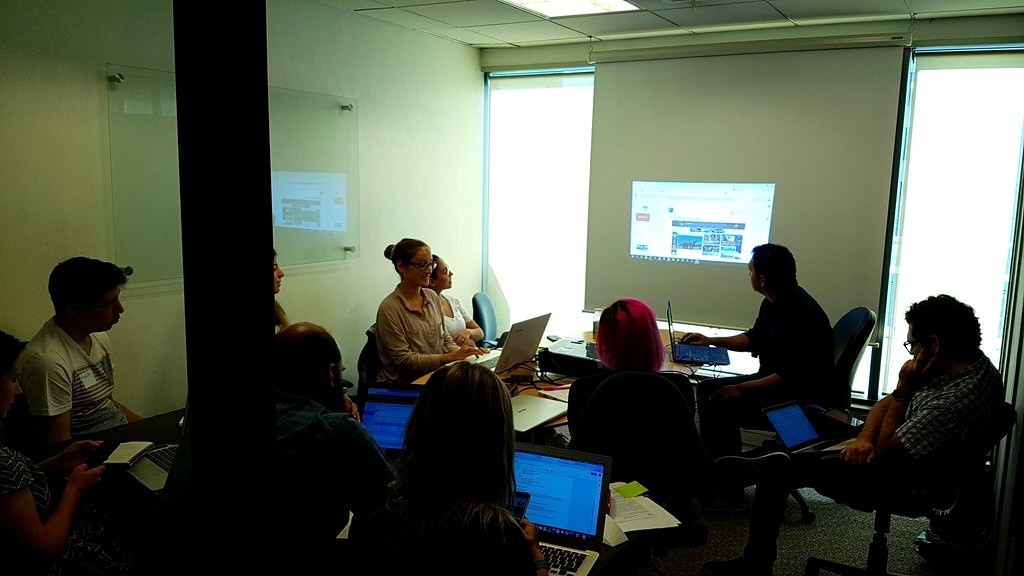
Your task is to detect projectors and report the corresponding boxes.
[538,338,606,378]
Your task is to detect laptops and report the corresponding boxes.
[667,299,731,366]
[506,441,612,576]
[357,382,424,462]
[761,401,859,456]
[125,398,190,494]
[446,313,551,374]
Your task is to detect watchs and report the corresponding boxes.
[892,390,909,402]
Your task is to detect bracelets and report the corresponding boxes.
[536,560,547,570]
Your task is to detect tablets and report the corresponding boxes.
[823,408,864,426]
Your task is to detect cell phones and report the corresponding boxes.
[547,336,561,342]
[509,491,530,524]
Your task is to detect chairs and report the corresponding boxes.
[472,292,499,349]
[763,306,877,524]
[804,402,1017,576]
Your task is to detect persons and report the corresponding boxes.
[276,323,399,576]
[0,330,132,576]
[376,237,490,382]
[4,257,144,444]
[272,251,288,332]
[341,361,548,576]
[681,244,837,509]
[566,299,696,447]
[704,293,1006,576]
[424,253,485,346]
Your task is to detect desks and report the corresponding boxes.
[23,317,763,576]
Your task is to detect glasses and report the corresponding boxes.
[403,262,437,270]
[903,338,928,354]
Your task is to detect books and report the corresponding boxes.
[51,439,155,467]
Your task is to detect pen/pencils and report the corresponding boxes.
[543,387,570,391]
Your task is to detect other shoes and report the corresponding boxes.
[698,488,747,512]
[711,447,791,482]
[703,555,771,576]
